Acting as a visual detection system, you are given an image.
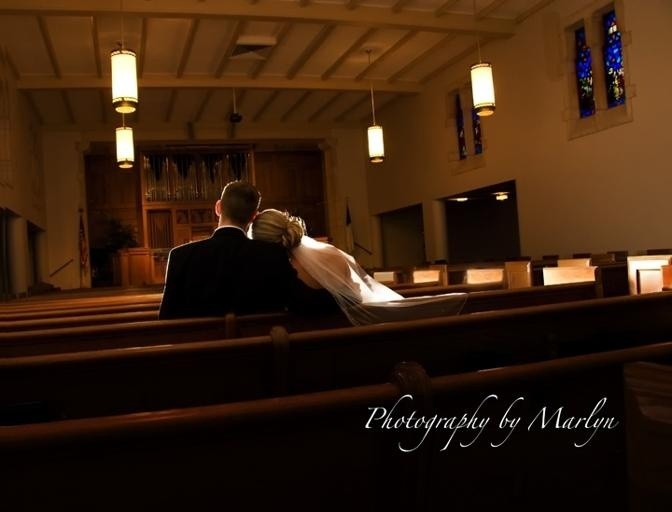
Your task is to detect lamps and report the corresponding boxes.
[106,2,142,170]
[493,190,509,203]
[464,1,497,118]
[362,49,387,165]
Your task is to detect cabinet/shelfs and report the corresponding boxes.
[113,144,255,290]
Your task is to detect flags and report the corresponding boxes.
[345,201,356,255]
[78,212,89,275]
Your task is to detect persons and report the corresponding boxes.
[248,209,357,290]
[157,180,364,320]
